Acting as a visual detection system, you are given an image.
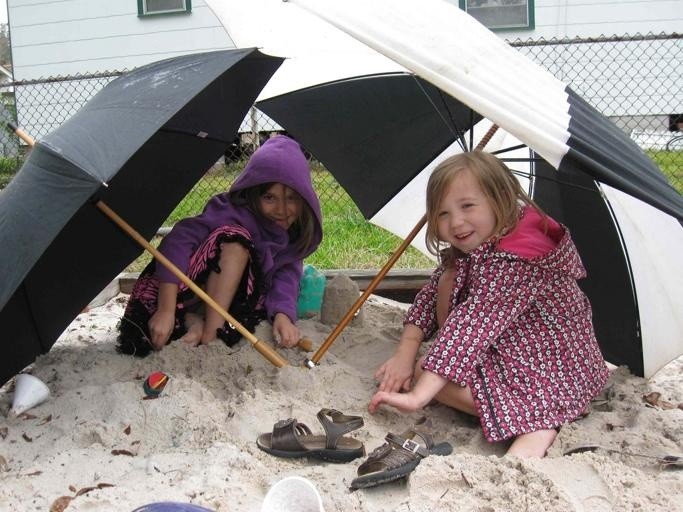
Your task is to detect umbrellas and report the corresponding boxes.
[1,46,319,394]
[200,0,683,382]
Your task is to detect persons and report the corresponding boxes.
[367,149,611,459]
[114,133,324,357]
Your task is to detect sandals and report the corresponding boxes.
[257,408,453,488]
